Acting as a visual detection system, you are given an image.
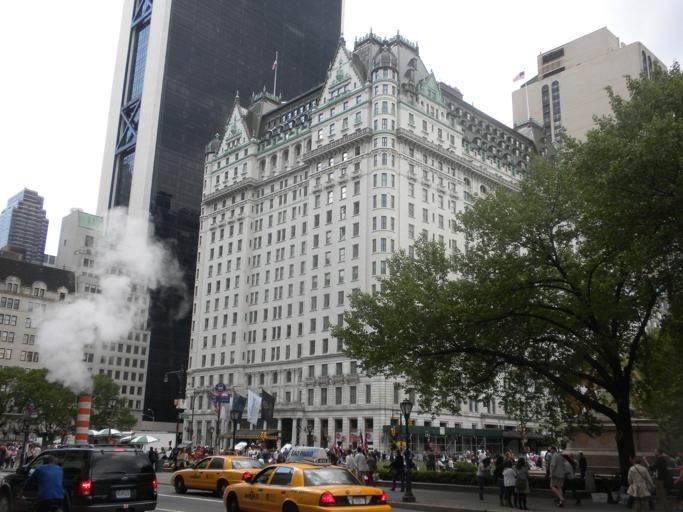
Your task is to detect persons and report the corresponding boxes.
[18,456,64,512]
[0,439,42,470]
[545,445,587,506]
[626,448,670,511]
[476,454,530,510]
[147,442,210,472]
[245,444,293,464]
[423,447,486,472]
[328,440,406,492]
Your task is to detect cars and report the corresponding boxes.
[220,447,393,511]
[165,454,264,492]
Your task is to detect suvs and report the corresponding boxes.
[0,441,157,511]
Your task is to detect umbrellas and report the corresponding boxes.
[87,428,158,444]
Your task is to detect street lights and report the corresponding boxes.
[145,408,154,432]
[396,397,416,502]
[162,361,185,451]
[229,407,240,454]
[304,422,315,448]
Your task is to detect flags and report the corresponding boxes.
[246,390,262,426]
[229,390,247,422]
[261,389,276,424]
[514,71,525,82]
[210,391,230,421]
[271,54,277,71]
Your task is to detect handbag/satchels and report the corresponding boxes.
[515,479,526,490]
[647,483,655,495]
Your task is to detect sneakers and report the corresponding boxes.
[556,499,564,506]
